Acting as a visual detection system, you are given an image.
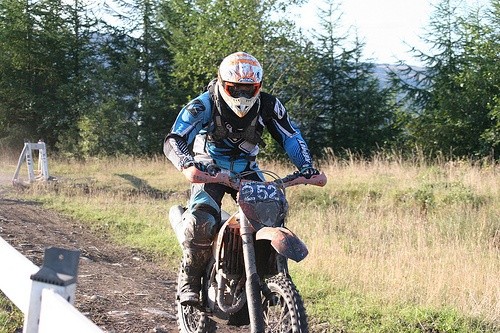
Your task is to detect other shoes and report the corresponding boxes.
[177,270,201,306]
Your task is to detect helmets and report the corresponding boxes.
[218,52,263,118]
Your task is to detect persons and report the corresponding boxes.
[164,51,320,306]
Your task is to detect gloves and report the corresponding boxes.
[182,161,206,172]
[301,167,319,179]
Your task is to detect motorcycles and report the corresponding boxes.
[169,164,327,333]
